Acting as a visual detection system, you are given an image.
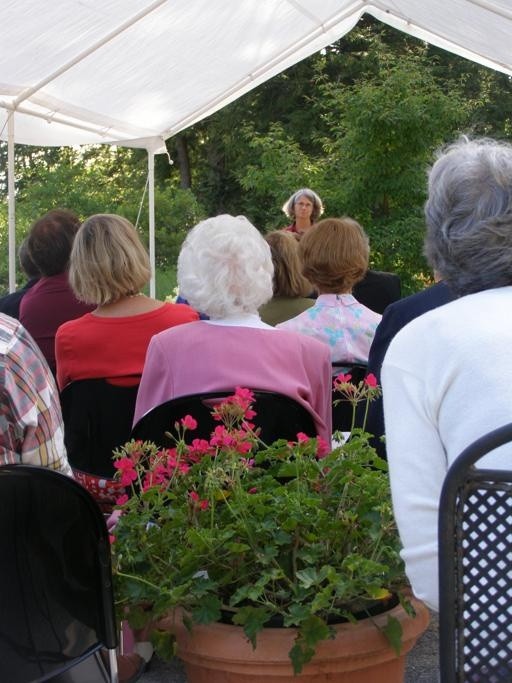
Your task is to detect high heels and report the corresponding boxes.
[131,641,153,672]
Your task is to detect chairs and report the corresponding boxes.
[1,464,121,683]
[332,360,368,436]
[130,387,317,449]
[436,422,511,681]
[59,373,142,478]
[352,270,402,318]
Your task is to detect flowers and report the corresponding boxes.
[102,371,425,674]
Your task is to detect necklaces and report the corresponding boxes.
[107,292,144,303]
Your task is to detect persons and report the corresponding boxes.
[55,213,200,388]
[380,133,512,674]
[176,295,210,320]
[278,188,325,241]
[270,219,384,378]
[0,238,42,319]
[108,212,332,549]
[258,230,316,326]
[371,261,470,461]
[0,314,76,484]
[19,209,98,383]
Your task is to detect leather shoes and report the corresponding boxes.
[99,651,145,683]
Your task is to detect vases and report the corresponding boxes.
[174,588,429,681]
